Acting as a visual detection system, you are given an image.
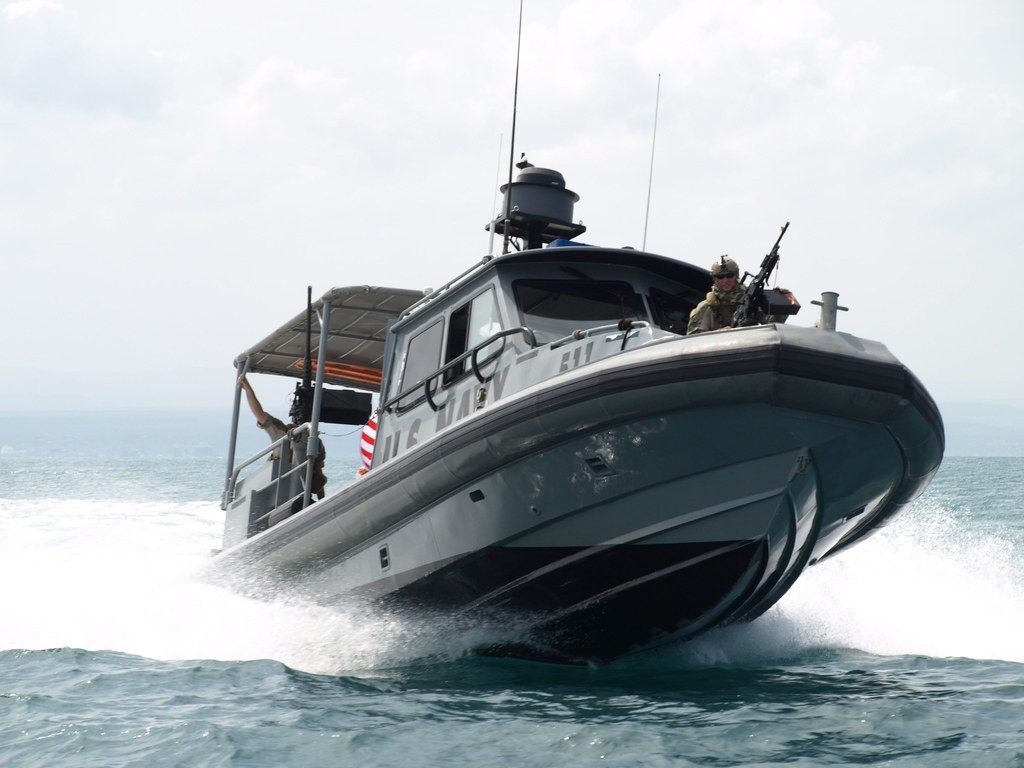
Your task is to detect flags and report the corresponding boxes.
[360,412,378,471]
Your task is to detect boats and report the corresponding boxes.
[193,0,946,674]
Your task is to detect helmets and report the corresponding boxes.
[709,257,738,276]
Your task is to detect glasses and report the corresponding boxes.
[713,273,735,279]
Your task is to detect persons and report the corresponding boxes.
[237,373,327,515]
[686,255,791,335]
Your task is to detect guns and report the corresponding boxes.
[730,222,801,328]
[288,287,374,428]
